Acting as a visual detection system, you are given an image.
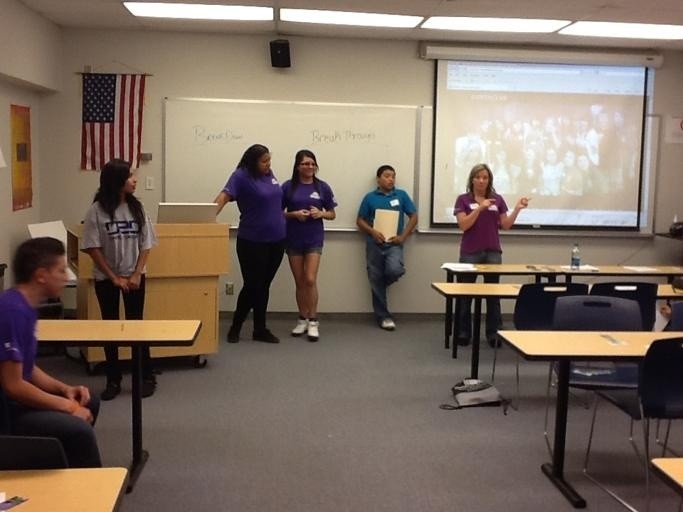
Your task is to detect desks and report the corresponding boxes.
[35,320,203,493]
[0,467,130,512]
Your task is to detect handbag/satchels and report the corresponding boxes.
[452,376,505,407]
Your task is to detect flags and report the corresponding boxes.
[81,72,145,171]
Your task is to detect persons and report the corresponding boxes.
[280,150,336,338]
[356,165,418,330]
[0,237,103,468]
[453,163,530,349]
[213,143,287,344]
[79,158,155,400]
[456,106,638,197]
[660,303,671,331]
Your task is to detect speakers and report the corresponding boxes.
[269,39,291,68]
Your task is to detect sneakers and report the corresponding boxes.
[253,328,279,343]
[291,318,308,336]
[381,318,395,329]
[458,337,469,346]
[307,320,320,341]
[490,340,501,348]
[227,323,240,343]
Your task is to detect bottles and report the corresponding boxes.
[571,243,580,271]
[673,214,678,224]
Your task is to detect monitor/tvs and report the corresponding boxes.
[157,203,218,224]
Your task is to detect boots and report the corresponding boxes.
[142,374,157,398]
[101,371,122,400]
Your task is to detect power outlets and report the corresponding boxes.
[226,283,234,295]
